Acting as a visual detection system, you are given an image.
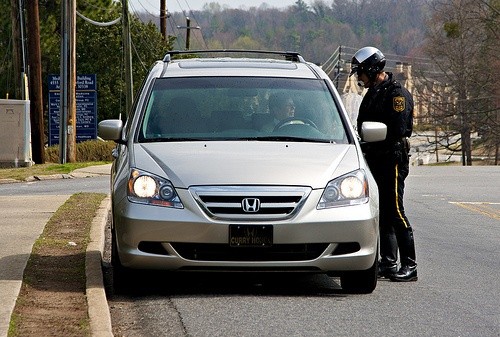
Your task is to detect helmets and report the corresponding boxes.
[349,46,386,88]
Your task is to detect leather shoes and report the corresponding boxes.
[390,264,418,281]
[377,262,396,278]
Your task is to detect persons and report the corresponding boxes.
[260,91,303,135]
[349,47,418,282]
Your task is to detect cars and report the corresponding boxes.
[99,50,388,294]
